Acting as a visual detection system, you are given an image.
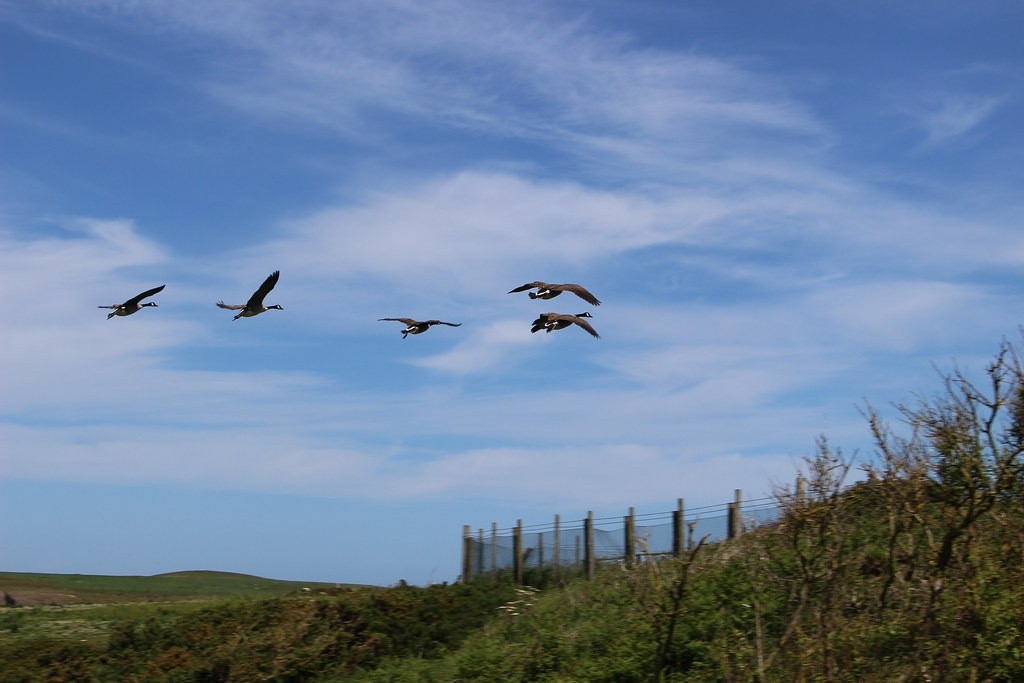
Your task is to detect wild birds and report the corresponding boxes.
[95,268,601,341]
